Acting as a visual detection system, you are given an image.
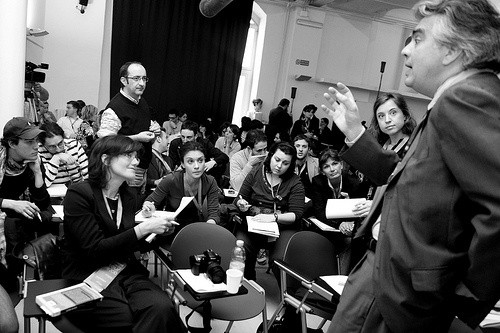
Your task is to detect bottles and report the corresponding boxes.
[228,240,246,286]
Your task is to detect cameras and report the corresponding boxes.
[190,248,226,284]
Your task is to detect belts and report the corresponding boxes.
[366,235,378,254]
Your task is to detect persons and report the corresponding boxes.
[96,62,161,196]
[0,81,371,332]
[351,92,418,245]
[321,0,500,333]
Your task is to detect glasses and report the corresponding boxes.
[44,142,64,150]
[125,76,148,82]
[119,153,140,163]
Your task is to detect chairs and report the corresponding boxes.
[259,230,340,333]
[20,231,60,333]
[164,221,269,333]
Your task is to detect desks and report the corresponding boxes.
[46,184,69,197]
[135,209,174,224]
[307,273,349,304]
[23,278,97,319]
[51,204,64,223]
[223,188,238,197]
[175,268,249,301]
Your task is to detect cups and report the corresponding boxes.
[226,269,243,294]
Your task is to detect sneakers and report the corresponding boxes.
[257,249,267,265]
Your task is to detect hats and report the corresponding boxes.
[3,117,44,139]
